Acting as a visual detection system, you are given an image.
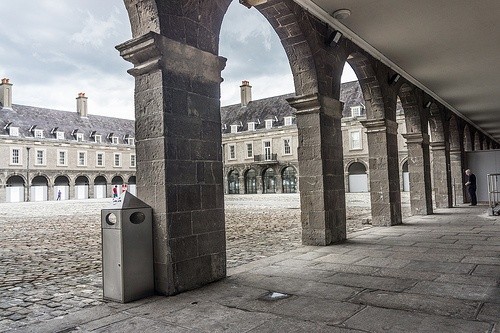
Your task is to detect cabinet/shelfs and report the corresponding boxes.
[487,173,500,217]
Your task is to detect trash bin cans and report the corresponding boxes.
[99,189,157,303]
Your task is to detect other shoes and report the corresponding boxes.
[469,204,477,206]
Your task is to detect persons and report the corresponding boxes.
[57,190,61,200]
[464,169,478,206]
[113,186,120,201]
